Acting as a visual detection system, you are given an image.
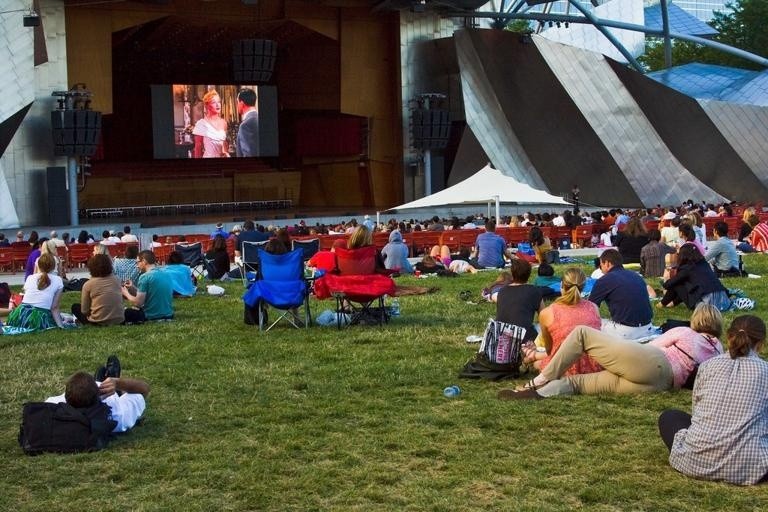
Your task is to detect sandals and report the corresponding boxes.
[519,343,536,370]
[525,339,538,352]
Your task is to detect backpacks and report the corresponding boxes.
[19,402,117,455]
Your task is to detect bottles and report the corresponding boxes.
[8,293,15,309]
[738,254,744,273]
[390,300,400,317]
[444,385,461,398]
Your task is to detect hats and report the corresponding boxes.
[17,230,24,237]
[50,230,58,238]
[108,230,117,236]
[266,225,275,232]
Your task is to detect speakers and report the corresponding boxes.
[431,156,445,194]
[412,108,452,151]
[410,4,425,13]
[45,165,71,229]
[24,16,40,26]
[230,37,278,83]
[51,110,103,157]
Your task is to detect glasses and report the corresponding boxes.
[135,260,142,264]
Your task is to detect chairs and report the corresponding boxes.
[246,249,325,329]
[618,223,625,230]
[460,230,475,251]
[162,245,174,264]
[104,245,117,257]
[236,241,271,289]
[116,242,127,257]
[201,240,215,254]
[474,229,486,239]
[554,225,571,249]
[426,231,442,255]
[292,238,320,260]
[441,231,460,256]
[0,247,12,274]
[69,244,89,269]
[509,227,530,246]
[88,243,96,259]
[372,233,386,246]
[723,218,739,238]
[126,243,138,259]
[703,218,722,237]
[225,238,235,263]
[592,224,608,234]
[10,242,30,274]
[151,247,163,264]
[736,215,744,232]
[327,246,394,330]
[412,231,426,256]
[757,213,768,223]
[645,222,659,232]
[539,227,553,246]
[576,226,592,247]
[664,219,672,226]
[54,246,67,271]
[318,235,345,249]
[496,227,509,246]
[401,234,413,258]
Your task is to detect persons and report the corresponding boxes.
[515,303,729,396]
[739,208,759,240]
[204,236,229,280]
[481,262,519,303]
[120,252,174,323]
[660,218,680,246]
[614,221,646,264]
[572,184,580,205]
[7,252,66,333]
[677,224,707,267]
[34,241,63,282]
[191,91,230,156]
[528,227,558,265]
[334,225,370,314]
[709,223,746,278]
[420,246,452,275]
[235,220,265,288]
[742,217,766,252]
[214,216,348,238]
[307,239,347,274]
[659,316,766,486]
[590,257,609,277]
[654,243,733,314]
[72,255,125,326]
[163,252,197,299]
[381,232,415,274]
[235,87,258,156]
[468,222,518,269]
[48,356,149,434]
[0,225,190,254]
[588,248,653,342]
[639,230,677,277]
[534,263,564,295]
[360,211,470,234]
[524,270,605,374]
[436,246,480,276]
[477,200,731,227]
[0,282,25,315]
[254,239,308,326]
[495,259,546,350]
[685,212,706,249]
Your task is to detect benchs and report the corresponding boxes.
[117,207,133,214]
[208,203,222,212]
[102,208,116,216]
[222,203,237,211]
[178,204,194,214]
[277,200,292,208]
[85,209,102,217]
[251,201,264,210]
[163,205,178,216]
[237,201,251,212]
[195,203,208,215]
[107,211,123,218]
[133,207,148,215]
[265,201,279,209]
[149,206,164,216]
[91,212,107,217]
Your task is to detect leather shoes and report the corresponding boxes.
[94,365,106,382]
[105,354,121,379]
[466,290,472,299]
[459,290,466,301]
[514,378,548,393]
